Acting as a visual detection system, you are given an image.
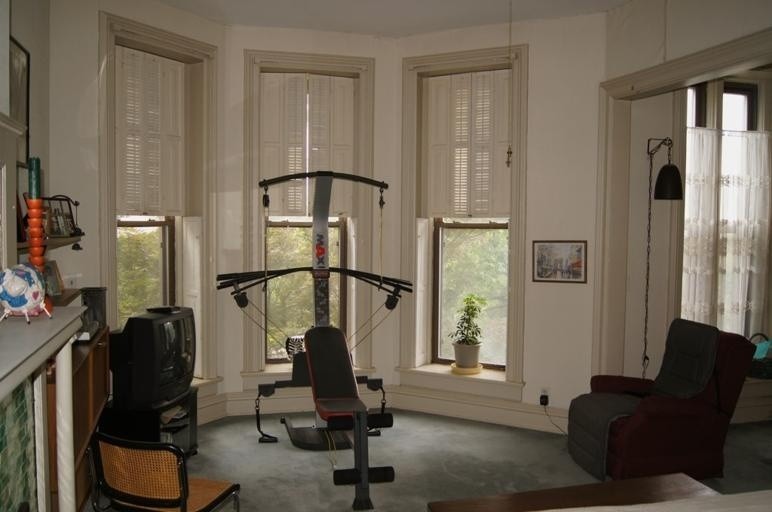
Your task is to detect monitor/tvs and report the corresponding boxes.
[109,307,196,411]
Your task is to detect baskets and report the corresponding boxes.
[747,332,772,380]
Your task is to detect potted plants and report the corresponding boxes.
[447,289,490,368]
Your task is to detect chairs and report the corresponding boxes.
[88,429,242,512]
[568,315,759,481]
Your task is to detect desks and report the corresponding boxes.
[104,386,199,459]
[427,470,719,512]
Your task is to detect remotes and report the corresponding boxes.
[147,307,173,313]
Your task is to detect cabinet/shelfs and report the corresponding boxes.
[46,325,110,511]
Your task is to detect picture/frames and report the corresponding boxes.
[39,196,78,238]
[8,32,35,170]
[530,238,589,285]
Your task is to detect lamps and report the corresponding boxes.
[640,133,686,379]
